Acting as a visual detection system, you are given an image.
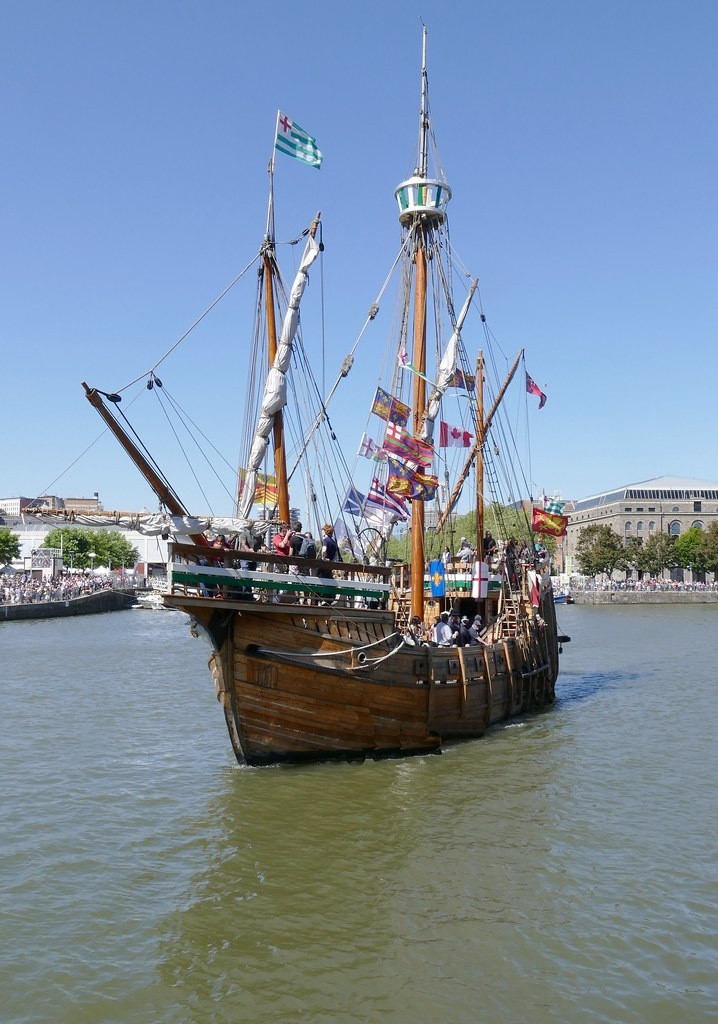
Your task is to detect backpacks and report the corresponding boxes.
[294,534,318,556]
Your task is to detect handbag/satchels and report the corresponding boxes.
[335,557,344,562]
[402,626,417,646]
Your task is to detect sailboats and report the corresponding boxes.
[19,25,569,778]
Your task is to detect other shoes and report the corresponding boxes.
[217,594,222,598]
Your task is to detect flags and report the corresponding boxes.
[429,561,446,597]
[274,109,324,170]
[471,561,490,599]
[531,507,571,537]
[445,367,476,391]
[543,498,566,516]
[526,372,547,409]
[399,353,477,400]
[527,570,541,609]
[331,386,441,558]
[238,467,289,505]
[438,419,475,448]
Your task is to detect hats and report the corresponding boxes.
[461,615,469,622]
[452,610,460,615]
[473,620,482,626]
[441,610,450,615]
[459,537,467,541]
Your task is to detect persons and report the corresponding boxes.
[394,611,491,684]
[552,577,718,591]
[0,571,167,606]
[179,522,337,607]
[444,530,549,591]
